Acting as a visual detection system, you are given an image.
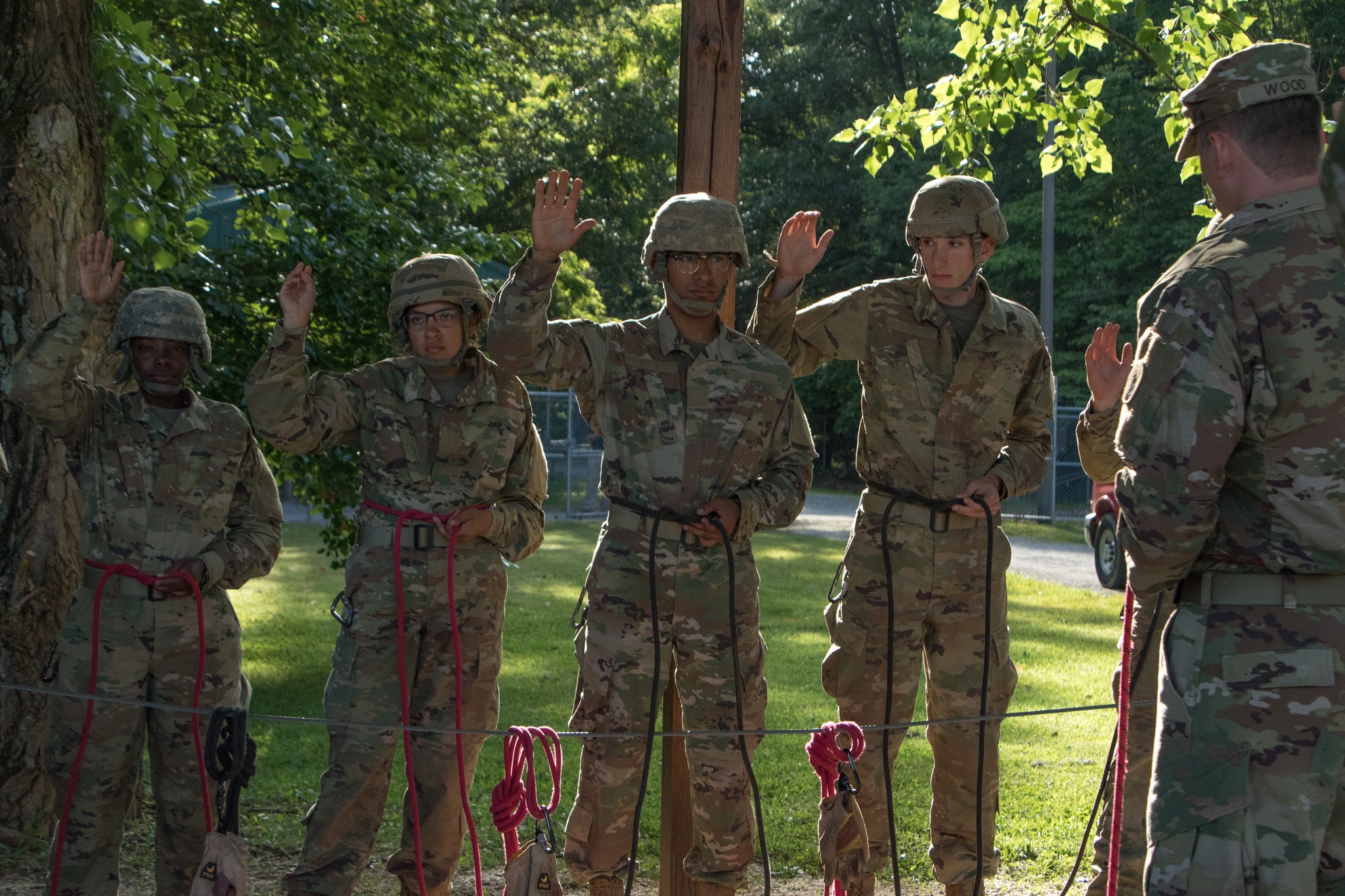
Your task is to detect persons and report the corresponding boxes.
[1075,50,1345,896]
[485,171,820,896]
[2,229,284,896]
[743,175,1054,895]
[242,250,547,896]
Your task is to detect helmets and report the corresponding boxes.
[641,192,750,269]
[388,252,494,345]
[905,175,1009,244]
[108,284,213,363]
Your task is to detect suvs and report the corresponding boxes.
[1083,478,1126,590]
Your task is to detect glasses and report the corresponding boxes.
[667,253,735,274]
[403,307,462,330]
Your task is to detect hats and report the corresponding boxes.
[1175,42,1316,163]
